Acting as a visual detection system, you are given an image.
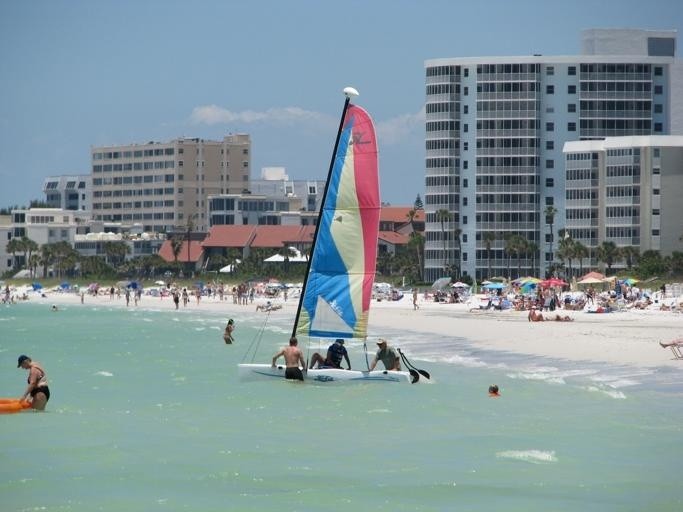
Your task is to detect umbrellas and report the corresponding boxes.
[450,281,470,289]
[481,280,493,285]
[510,270,639,294]
[482,282,507,289]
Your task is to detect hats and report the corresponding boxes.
[18,355,27,368]
[335,339,344,347]
[376,337,386,345]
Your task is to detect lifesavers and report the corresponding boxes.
[0,398,31,413]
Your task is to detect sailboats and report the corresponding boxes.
[234,85,412,384]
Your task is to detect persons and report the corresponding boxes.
[368,336,402,372]
[410,288,419,311]
[486,383,499,398]
[222,317,235,345]
[0,281,289,314]
[15,354,50,410]
[423,284,682,322]
[309,339,352,370]
[658,337,682,349]
[270,336,306,384]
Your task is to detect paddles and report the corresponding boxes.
[397,348,419,384]
[402,352,430,380]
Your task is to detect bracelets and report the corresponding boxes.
[23,391,28,398]
